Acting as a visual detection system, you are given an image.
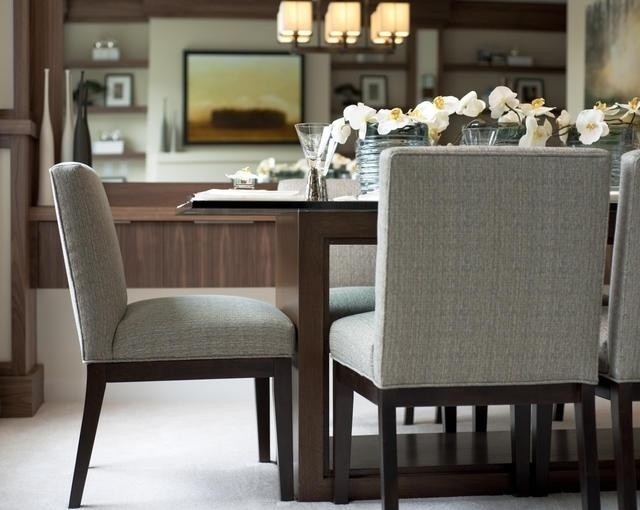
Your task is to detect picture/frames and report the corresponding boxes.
[517,76,545,105]
[104,73,133,106]
[360,74,388,109]
[183,50,305,145]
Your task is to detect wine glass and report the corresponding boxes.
[298,122,338,201]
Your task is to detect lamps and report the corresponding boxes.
[276,0,410,52]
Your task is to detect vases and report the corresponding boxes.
[461,126,525,146]
[357,125,427,195]
[571,126,637,187]
[36,67,94,208]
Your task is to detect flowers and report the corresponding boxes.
[488,87,610,147]
[593,97,640,129]
[332,91,483,141]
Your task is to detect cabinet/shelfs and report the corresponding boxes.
[65,61,148,161]
